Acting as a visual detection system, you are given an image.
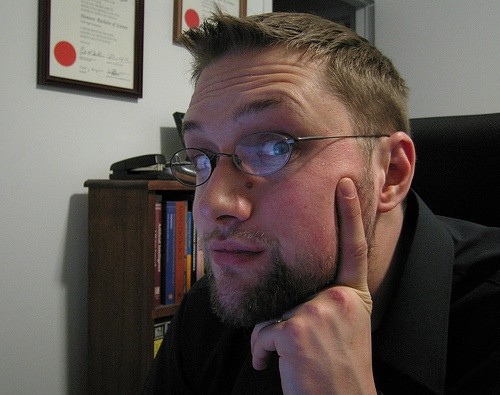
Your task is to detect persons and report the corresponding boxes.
[138,4,500,395]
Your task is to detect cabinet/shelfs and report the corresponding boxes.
[83,178,206,394]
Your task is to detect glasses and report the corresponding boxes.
[166,132,390,188]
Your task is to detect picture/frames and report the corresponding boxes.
[38,2,145,99]
[173,0,246,45]
